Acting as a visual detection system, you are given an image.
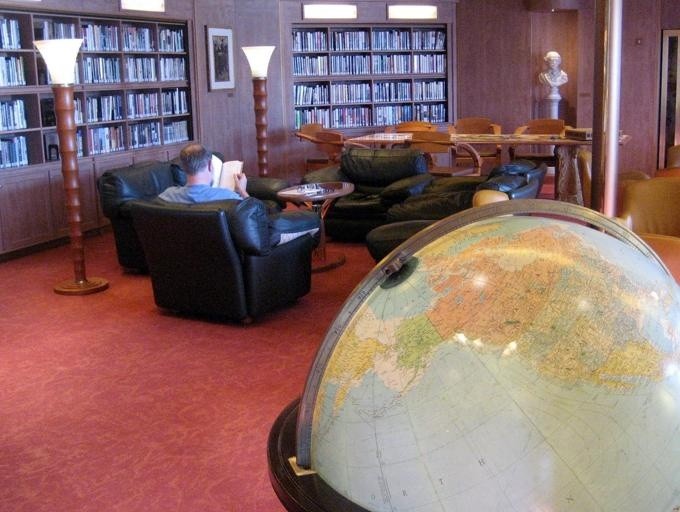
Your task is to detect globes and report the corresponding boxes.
[264,197,678,510]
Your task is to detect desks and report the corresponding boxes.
[267,396,364,510]
[348,133,632,202]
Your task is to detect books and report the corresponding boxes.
[0,15,190,169]
[291,30,327,51]
[331,30,370,51]
[565,127,624,140]
[373,54,411,74]
[413,30,445,50]
[372,29,410,51]
[293,55,328,76]
[413,54,446,74]
[294,81,447,131]
[331,54,371,76]
[208,153,244,193]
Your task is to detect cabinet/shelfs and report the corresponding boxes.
[291,22,453,137]
[0,11,200,255]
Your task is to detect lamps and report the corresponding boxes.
[240,45,277,178]
[31,38,109,296]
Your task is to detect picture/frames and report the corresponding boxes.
[656,28,680,169]
[204,22,238,93]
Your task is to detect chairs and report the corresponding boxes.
[410,132,481,177]
[409,158,548,199]
[300,148,434,243]
[654,144,680,177]
[316,131,370,158]
[162,151,291,213]
[96,165,187,276]
[448,116,502,165]
[295,123,356,155]
[508,118,572,168]
[621,176,679,239]
[126,194,323,326]
[576,150,650,219]
[366,178,539,265]
[384,122,439,133]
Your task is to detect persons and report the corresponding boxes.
[156,143,308,215]
[538,50,569,88]
